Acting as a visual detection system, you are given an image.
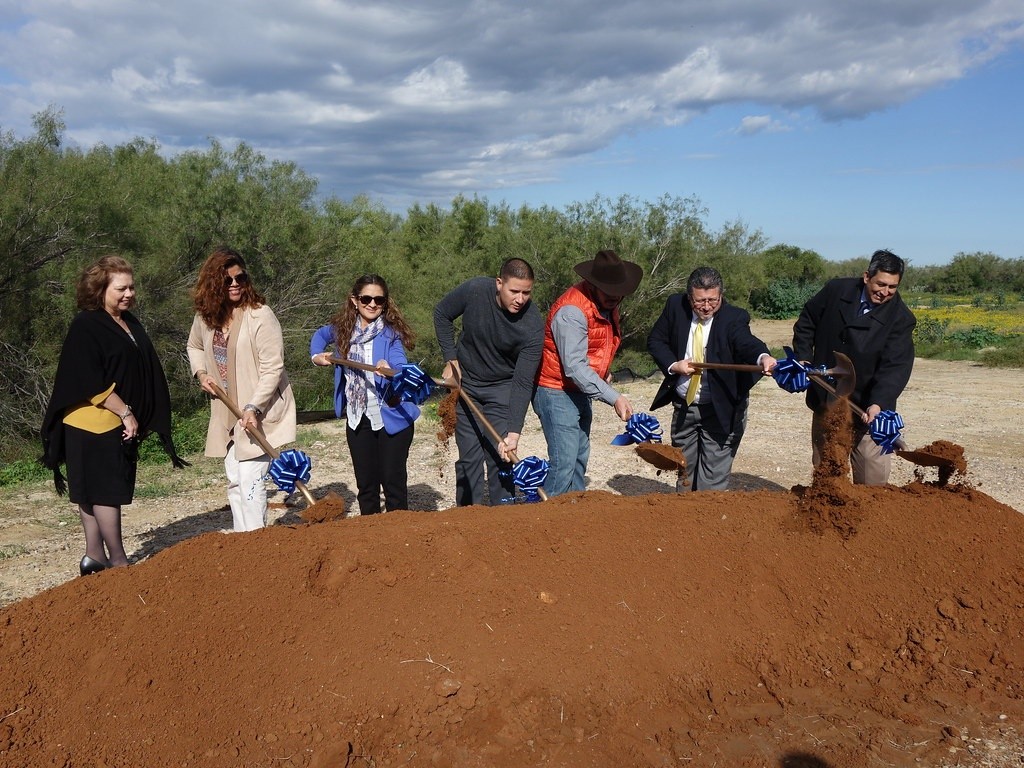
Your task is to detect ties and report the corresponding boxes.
[686,319,704,406]
[858,301,870,318]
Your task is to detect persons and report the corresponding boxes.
[792,250,917,485]
[531,249,643,499]
[434,258,545,507]
[311,274,421,516]
[186,247,297,532]
[33,254,192,577]
[647,268,778,493]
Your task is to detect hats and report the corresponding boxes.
[574,250,643,296]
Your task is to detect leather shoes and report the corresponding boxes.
[80,556,110,576]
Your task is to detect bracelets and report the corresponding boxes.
[196,371,208,383]
[120,405,132,419]
[243,404,260,415]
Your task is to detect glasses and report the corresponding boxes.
[691,295,720,306]
[223,273,249,285]
[356,295,386,305]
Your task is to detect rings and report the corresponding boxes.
[133,433,137,436]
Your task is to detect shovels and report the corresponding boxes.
[459,391,550,502]
[628,415,687,475]
[209,384,348,525]
[803,366,966,468]
[326,355,462,405]
[687,353,856,406]
